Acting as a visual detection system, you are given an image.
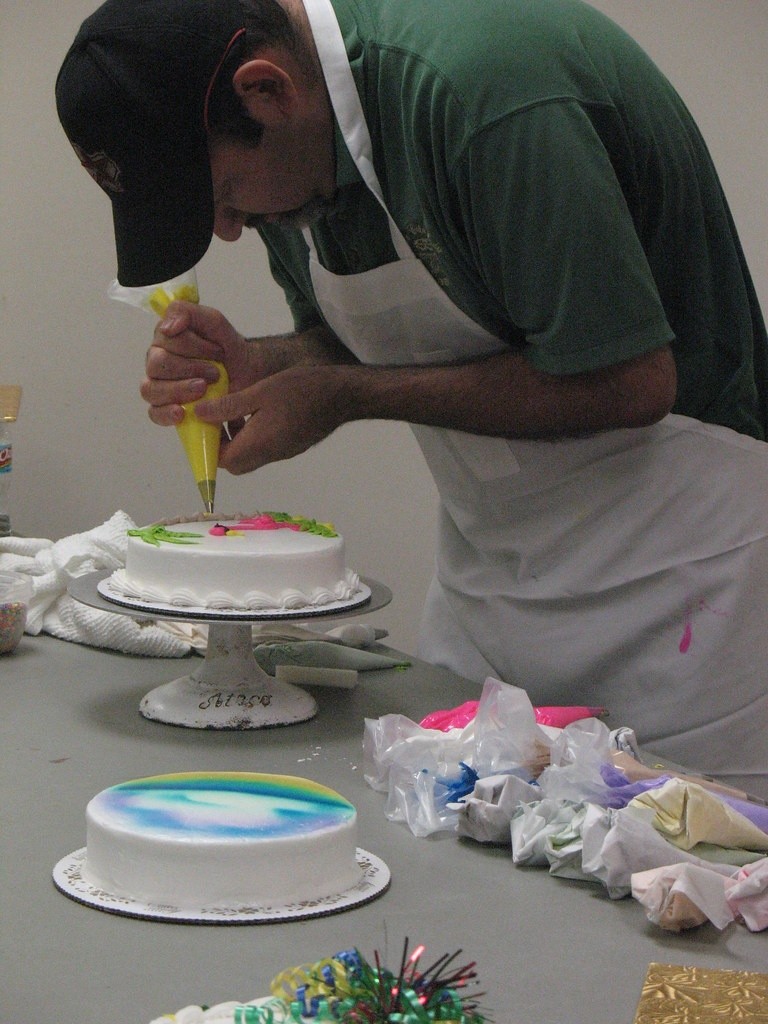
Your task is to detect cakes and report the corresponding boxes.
[81,772,360,912]
[108,509,360,609]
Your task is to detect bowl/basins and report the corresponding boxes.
[0,571,36,655]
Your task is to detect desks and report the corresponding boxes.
[1,624,767,1024]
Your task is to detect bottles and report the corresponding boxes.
[0,385,22,539]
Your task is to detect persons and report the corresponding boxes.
[54,0,768,803]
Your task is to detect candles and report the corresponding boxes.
[275,665,358,689]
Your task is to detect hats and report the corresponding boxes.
[55,0,233,287]
